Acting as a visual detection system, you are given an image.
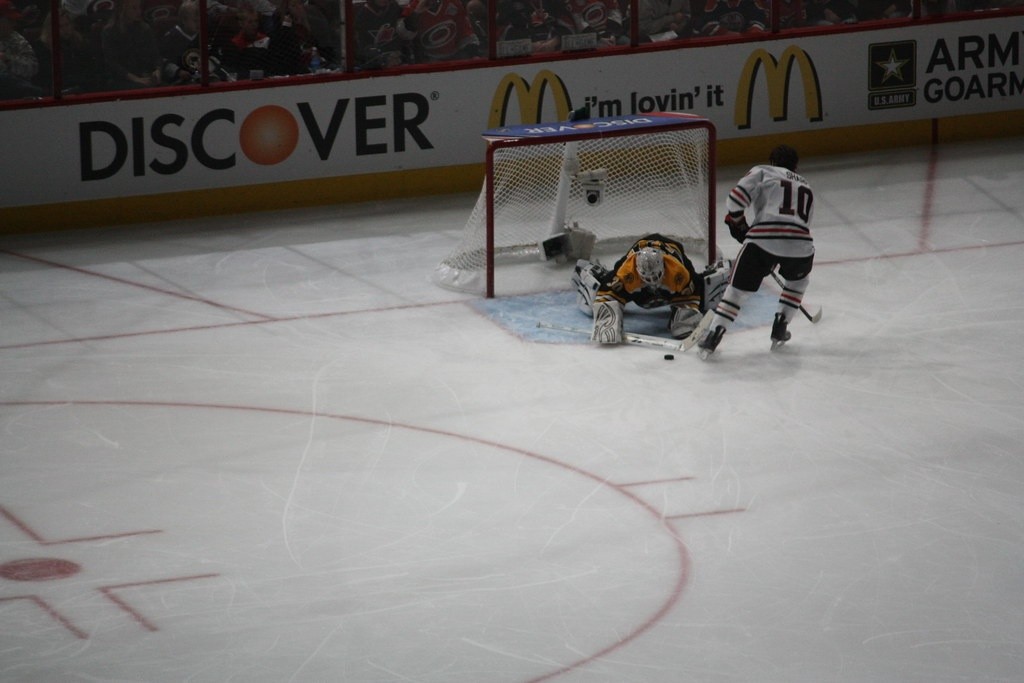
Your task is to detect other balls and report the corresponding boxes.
[664,354,674,360]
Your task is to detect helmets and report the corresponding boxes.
[635,247,665,288]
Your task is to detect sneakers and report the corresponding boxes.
[769,312,791,351]
[696,325,726,361]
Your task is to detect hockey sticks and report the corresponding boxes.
[771,271,822,323]
[535,308,717,352]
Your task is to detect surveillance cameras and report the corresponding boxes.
[583,182,604,208]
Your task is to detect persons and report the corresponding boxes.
[569,231,704,346]
[697,142,815,361]
[0,0,1024,94]
